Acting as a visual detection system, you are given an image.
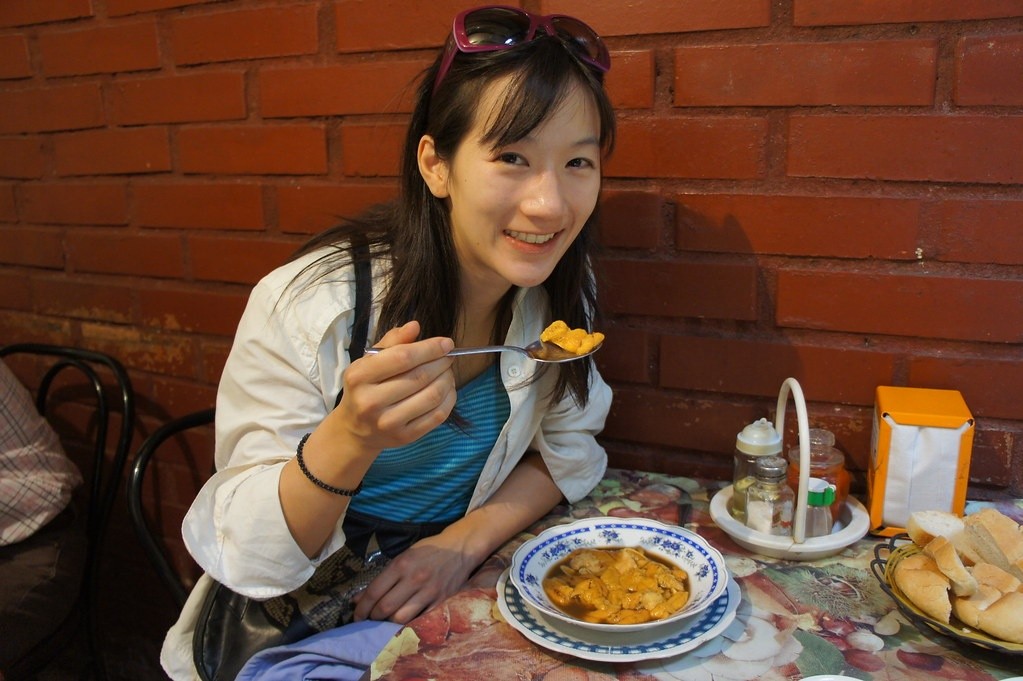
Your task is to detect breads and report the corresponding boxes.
[894,506,1023,644]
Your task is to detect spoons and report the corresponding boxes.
[362,341,605,363]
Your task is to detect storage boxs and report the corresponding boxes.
[867,385,976,539]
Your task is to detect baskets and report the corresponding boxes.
[872,532,1023,654]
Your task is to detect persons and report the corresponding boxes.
[0,359,94,675]
[160,4,617,681]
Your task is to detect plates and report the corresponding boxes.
[495,567,742,663]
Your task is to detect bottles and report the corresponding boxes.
[733,418,847,536]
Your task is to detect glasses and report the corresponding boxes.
[428,5,612,104]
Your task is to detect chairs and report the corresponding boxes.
[130,410,217,614]
[0,342,132,681]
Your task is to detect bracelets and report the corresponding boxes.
[297,434,363,495]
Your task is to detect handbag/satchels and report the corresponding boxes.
[193,508,467,681]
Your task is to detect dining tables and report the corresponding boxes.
[355,467,1023,681]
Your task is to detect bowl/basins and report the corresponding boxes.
[510,516,728,631]
